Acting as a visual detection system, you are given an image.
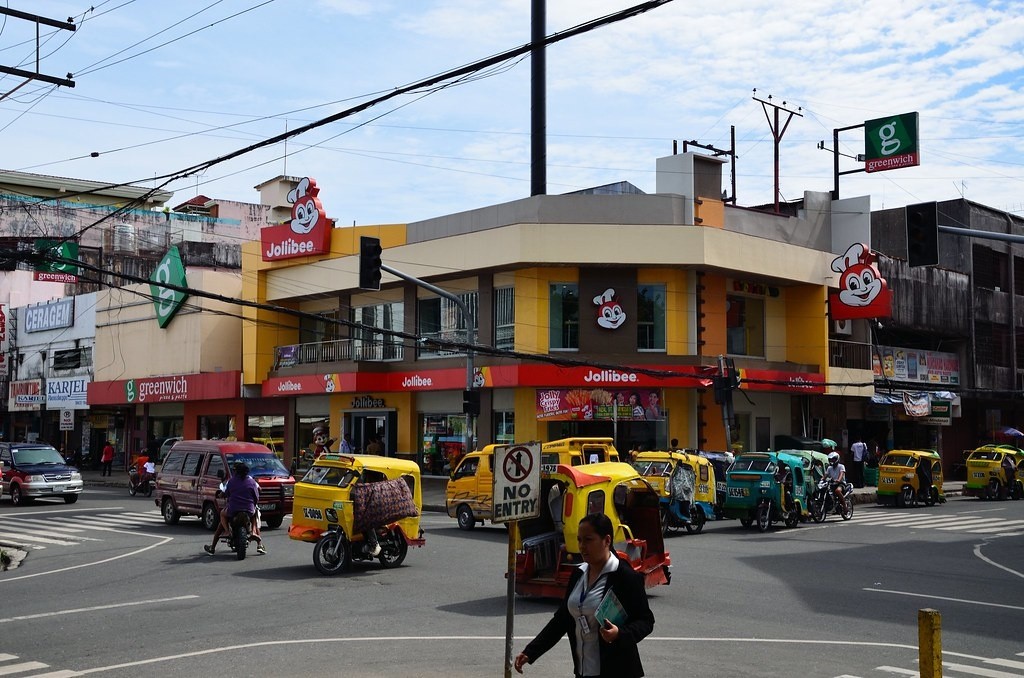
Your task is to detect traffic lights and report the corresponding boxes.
[726,357,741,389]
[359,236,382,292]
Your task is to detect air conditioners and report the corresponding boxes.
[830,319,852,335]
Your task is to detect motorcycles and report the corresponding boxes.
[961,444,1024,501]
[777,449,830,498]
[724,451,806,533]
[632,451,717,538]
[216,469,255,561]
[288,452,422,576]
[808,478,855,524]
[693,448,735,521]
[129,463,156,498]
[503,460,671,602]
[876,449,943,507]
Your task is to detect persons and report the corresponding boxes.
[821,452,849,514]
[667,459,690,523]
[913,458,931,499]
[339,433,355,455]
[130,448,150,486]
[671,438,679,452]
[358,475,383,556]
[646,391,662,420]
[850,434,867,489]
[101,441,114,477]
[615,392,624,406]
[775,463,793,514]
[368,435,386,456]
[514,512,655,678]
[1005,456,1016,492]
[628,393,645,421]
[204,462,267,556]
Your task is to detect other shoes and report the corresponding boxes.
[372,541,382,556]
[138,482,141,486]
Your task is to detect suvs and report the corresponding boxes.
[0,442,83,507]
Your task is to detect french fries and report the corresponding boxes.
[564,388,613,406]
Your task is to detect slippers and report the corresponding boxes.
[251,533,261,541]
[219,533,232,537]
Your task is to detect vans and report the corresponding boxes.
[153,439,295,533]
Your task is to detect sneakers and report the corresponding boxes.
[256,544,267,554]
[203,545,215,556]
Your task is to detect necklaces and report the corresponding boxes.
[589,570,603,581]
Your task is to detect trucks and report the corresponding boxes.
[445,436,620,532]
[158,436,182,466]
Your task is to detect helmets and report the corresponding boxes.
[828,452,840,466]
[141,448,148,456]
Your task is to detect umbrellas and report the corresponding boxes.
[987,426,1024,441]
